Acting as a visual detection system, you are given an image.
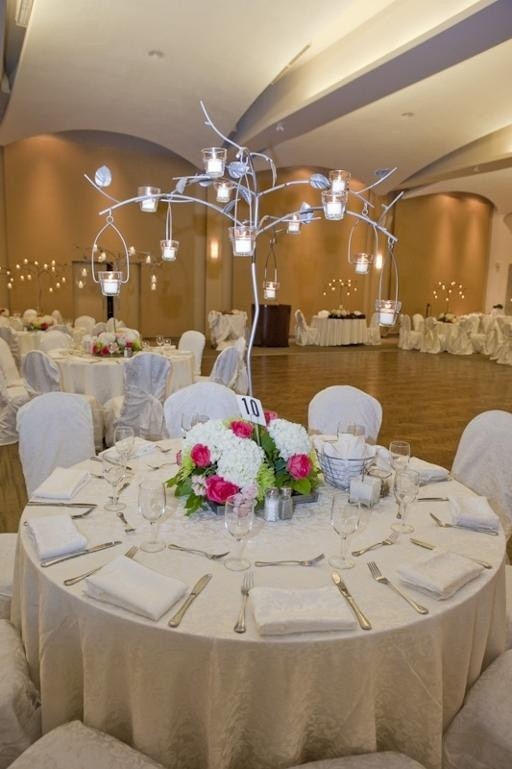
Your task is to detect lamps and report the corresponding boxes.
[432,280,464,321]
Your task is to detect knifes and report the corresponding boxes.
[410,538,494,570]
[329,571,372,632]
[40,541,124,569]
[28,501,98,508]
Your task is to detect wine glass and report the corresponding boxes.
[103,450,127,511]
[113,425,135,486]
[137,478,167,553]
[390,467,421,534]
[60,335,171,356]
[223,495,255,572]
[389,441,411,471]
[327,490,361,570]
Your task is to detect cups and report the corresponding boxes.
[335,421,356,441]
[349,423,366,445]
[181,416,197,438]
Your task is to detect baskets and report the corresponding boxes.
[315,447,376,491]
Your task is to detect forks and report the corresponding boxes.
[168,543,230,561]
[63,544,138,586]
[430,513,499,536]
[351,532,400,557]
[255,551,325,568]
[109,482,130,501]
[366,562,430,614]
[115,511,136,533]
